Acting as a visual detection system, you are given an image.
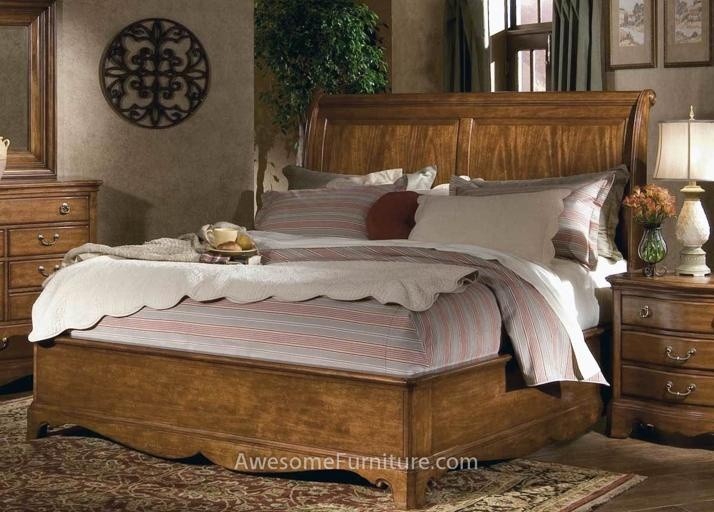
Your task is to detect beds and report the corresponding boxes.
[26,86,657,511]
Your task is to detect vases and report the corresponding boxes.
[0,136,11,179]
[637,227,667,278]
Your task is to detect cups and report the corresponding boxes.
[203,228,239,248]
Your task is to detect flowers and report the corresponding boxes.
[621,183,678,255]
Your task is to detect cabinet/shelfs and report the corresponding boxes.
[0,178,104,390]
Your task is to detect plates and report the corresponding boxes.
[206,245,258,257]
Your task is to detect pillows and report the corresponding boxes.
[455,170,616,269]
[326,167,405,189]
[448,164,630,262]
[253,175,408,238]
[407,188,571,266]
[365,189,421,240]
[282,164,438,191]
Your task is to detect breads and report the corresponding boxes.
[217,241,243,250]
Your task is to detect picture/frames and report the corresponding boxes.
[0,0,58,183]
[604,0,658,70]
[663,0,713,67]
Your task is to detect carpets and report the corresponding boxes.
[0,383,649,512]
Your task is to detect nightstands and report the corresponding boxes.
[604,270,714,438]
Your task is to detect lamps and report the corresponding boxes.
[652,105,714,278]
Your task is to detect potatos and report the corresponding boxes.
[236,236,253,249]
